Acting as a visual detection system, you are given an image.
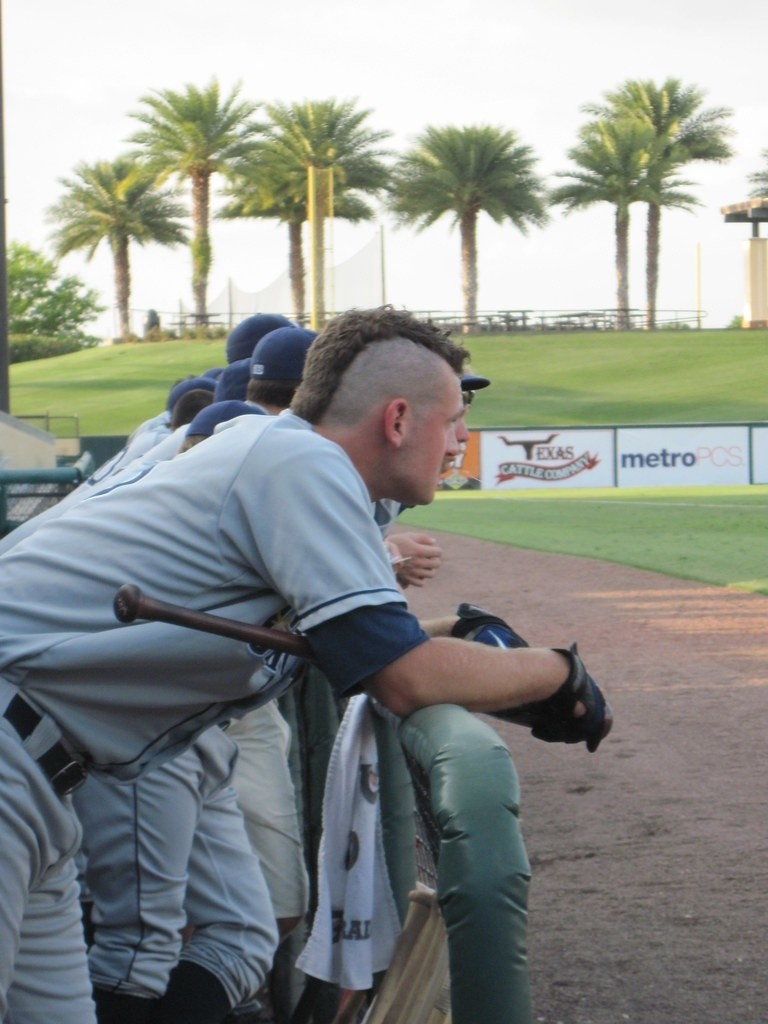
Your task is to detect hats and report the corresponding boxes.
[459,373,493,392]
[163,377,217,412]
[183,398,266,442]
[210,356,255,404]
[225,315,300,365]
[202,368,225,382]
[250,325,318,378]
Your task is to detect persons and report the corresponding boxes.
[1,313,494,1024]
[1,304,616,1024]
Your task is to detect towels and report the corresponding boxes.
[295,694,403,993]
